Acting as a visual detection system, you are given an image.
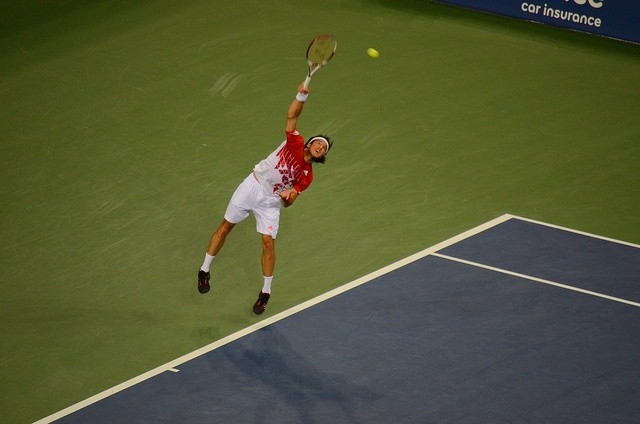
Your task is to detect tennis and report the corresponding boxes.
[367,48,379,58]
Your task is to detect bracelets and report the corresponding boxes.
[295,92,308,102]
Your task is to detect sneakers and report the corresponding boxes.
[198,270,211,295]
[254,292,271,316]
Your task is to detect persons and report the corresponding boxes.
[197,81,333,315]
